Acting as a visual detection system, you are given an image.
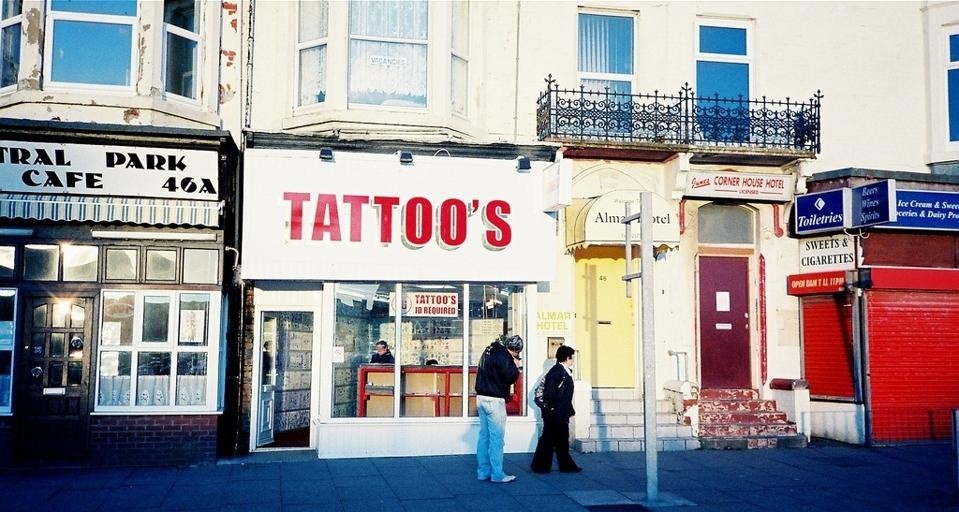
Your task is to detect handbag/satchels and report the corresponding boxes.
[534,377,548,410]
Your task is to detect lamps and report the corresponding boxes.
[399,150,415,165]
[319,146,332,161]
[517,154,531,171]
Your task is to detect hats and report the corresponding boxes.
[499,333,523,352]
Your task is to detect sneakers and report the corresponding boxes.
[491,475,515,482]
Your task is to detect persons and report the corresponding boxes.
[475,335,524,483]
[531,346,582,473]
[262,341,272,384]
[370,341,394,363]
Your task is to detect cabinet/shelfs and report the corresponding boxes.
[357,366,522,417]
